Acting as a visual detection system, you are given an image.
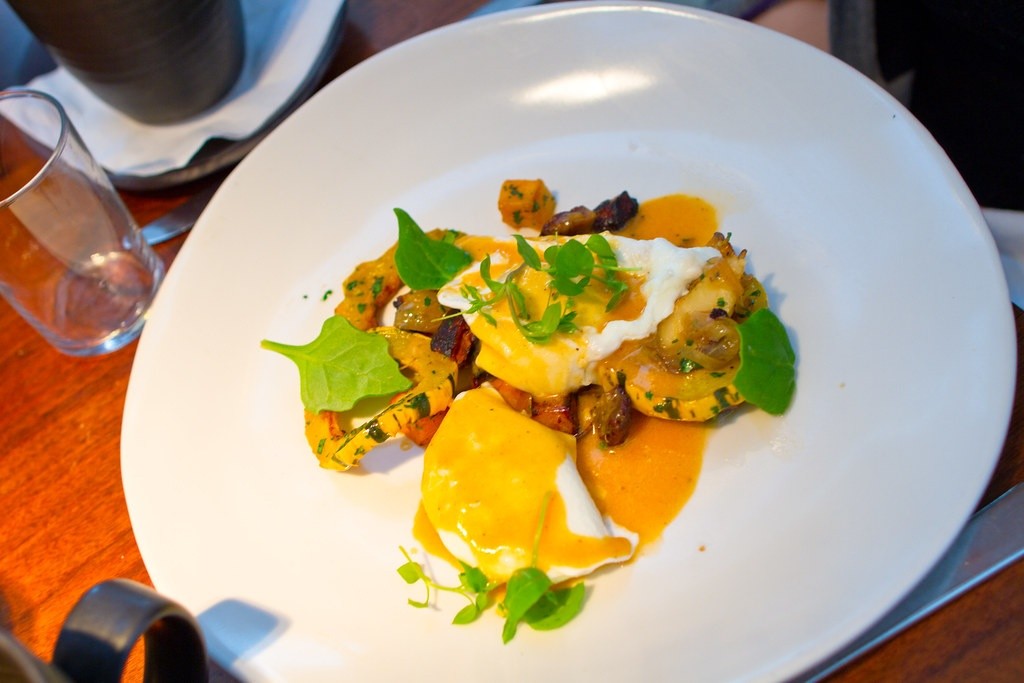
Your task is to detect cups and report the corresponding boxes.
[7,0,246,128]
[0,88,168,359]
[1,573,211,683]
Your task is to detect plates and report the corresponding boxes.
[116,0,1020,683]
[0,0,350,190]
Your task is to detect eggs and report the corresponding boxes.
[404,382,644,593]
[436,230,721,395]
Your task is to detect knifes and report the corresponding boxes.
[785,482,1024,683]
[121,185,216,251]
